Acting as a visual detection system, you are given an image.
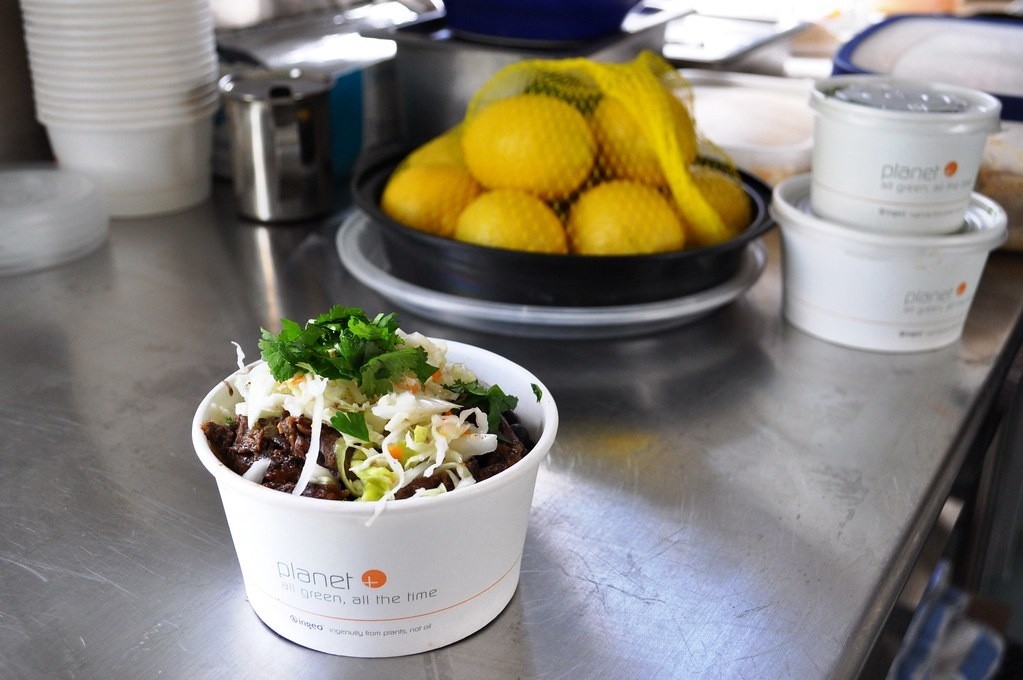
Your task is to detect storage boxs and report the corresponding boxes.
[356,0,700,151]
[831,14,1023,247]
[672,68,816,187]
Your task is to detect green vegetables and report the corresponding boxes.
[259,307,517,443]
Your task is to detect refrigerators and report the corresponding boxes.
[214,0,442,185]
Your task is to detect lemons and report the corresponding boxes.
[383,80,749,253]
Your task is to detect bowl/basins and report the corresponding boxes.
[641,69,815,173]
[806,73,1002,237]
[771,171,1008,354]
[442,0,643,48]
[21,0,222,221]
[192,337,559,658]
[350,144,779,307]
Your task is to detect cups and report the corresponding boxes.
[219,68,336,221]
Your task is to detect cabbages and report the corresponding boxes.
[212,327,498,527]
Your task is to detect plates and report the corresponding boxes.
[336,205,768,339]
